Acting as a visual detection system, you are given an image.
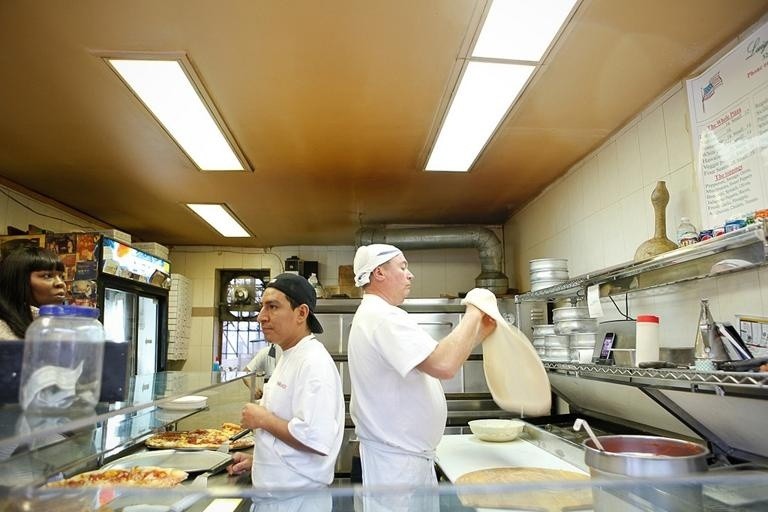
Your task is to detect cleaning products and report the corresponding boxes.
[211,356,220,385]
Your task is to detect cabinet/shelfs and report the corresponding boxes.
[515,222,768,462]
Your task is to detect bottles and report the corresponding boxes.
[635,315,659,369]
[17,302,104,417]
[693,297,730,371]
[676,217,696,243]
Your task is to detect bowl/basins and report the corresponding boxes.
[528,258,570,293]
[529,305,601,364]
[582,433,712,475]
[467,418,524,443]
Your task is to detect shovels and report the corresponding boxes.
[215,428,252,454]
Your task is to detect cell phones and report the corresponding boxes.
[600,332,616,358]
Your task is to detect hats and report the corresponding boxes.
[267,273,324,333]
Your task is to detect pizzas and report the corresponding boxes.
[42,465,190,489]
[145,422,254,450]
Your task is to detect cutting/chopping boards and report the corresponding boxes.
[451,466,592,512]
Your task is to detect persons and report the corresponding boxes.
[345,242,498,511]
[0,238,67,340]
[227,272,345,511]
[240,338,285,401]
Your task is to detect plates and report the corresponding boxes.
[157,396,207,411]
[95,227,166,261]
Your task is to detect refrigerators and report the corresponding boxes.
[94,240,170,376]
[94,376,166,457]
